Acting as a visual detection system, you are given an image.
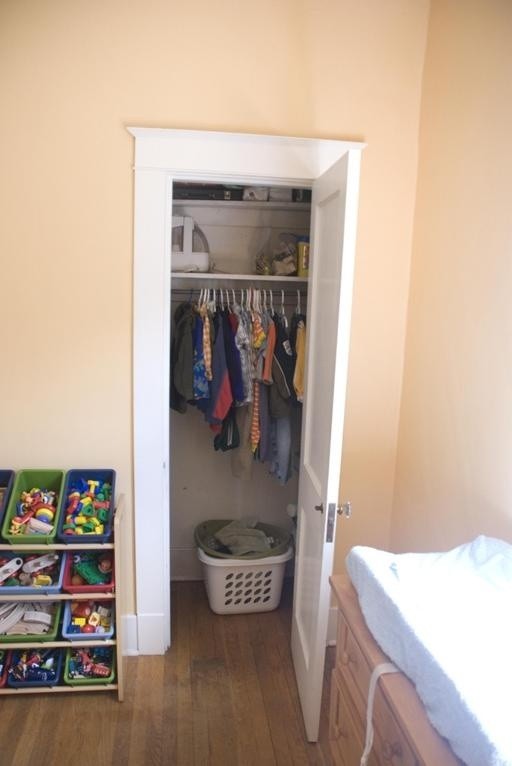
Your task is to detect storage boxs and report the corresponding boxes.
[1,468,115,687]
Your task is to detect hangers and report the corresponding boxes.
[175,288,304,330]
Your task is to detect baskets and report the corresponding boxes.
[194,520,294,615]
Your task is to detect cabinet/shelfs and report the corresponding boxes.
[170,198,309,281]
[326,572,467,765]
[0,544,125,701]
